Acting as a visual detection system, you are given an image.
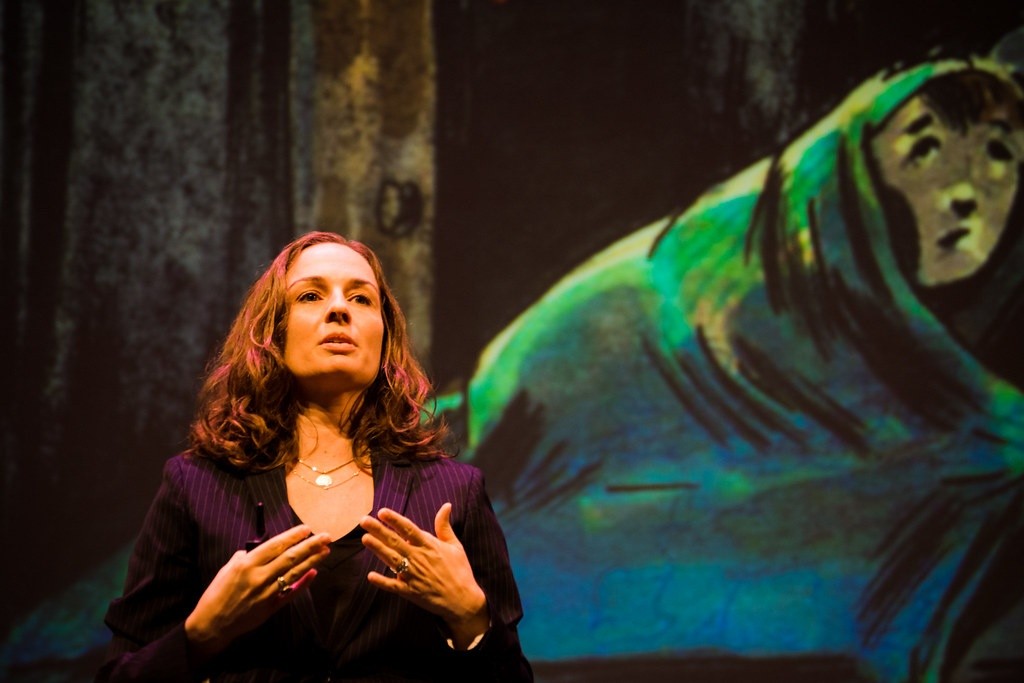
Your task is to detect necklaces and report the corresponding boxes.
[292,448,371,490]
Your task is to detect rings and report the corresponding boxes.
[390,559,408,573]
[277,576,292,593]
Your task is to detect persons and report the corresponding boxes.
[105,232,535,683]
